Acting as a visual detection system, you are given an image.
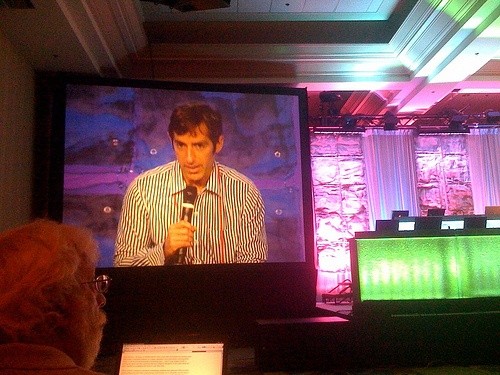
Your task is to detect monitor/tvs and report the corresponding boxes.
[30,67,316,323]
[116,336,229,375]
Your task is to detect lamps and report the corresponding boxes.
[447,113,469,132]
[319,91,342,103]
[382,113,399,131]
[344,113,357,130]
[484,109,500,125]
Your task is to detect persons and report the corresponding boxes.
[113,102,267,264]
[0,218,107,375]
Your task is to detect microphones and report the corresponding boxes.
[173,187,197,263]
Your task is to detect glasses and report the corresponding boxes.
[79,275,112,293]
